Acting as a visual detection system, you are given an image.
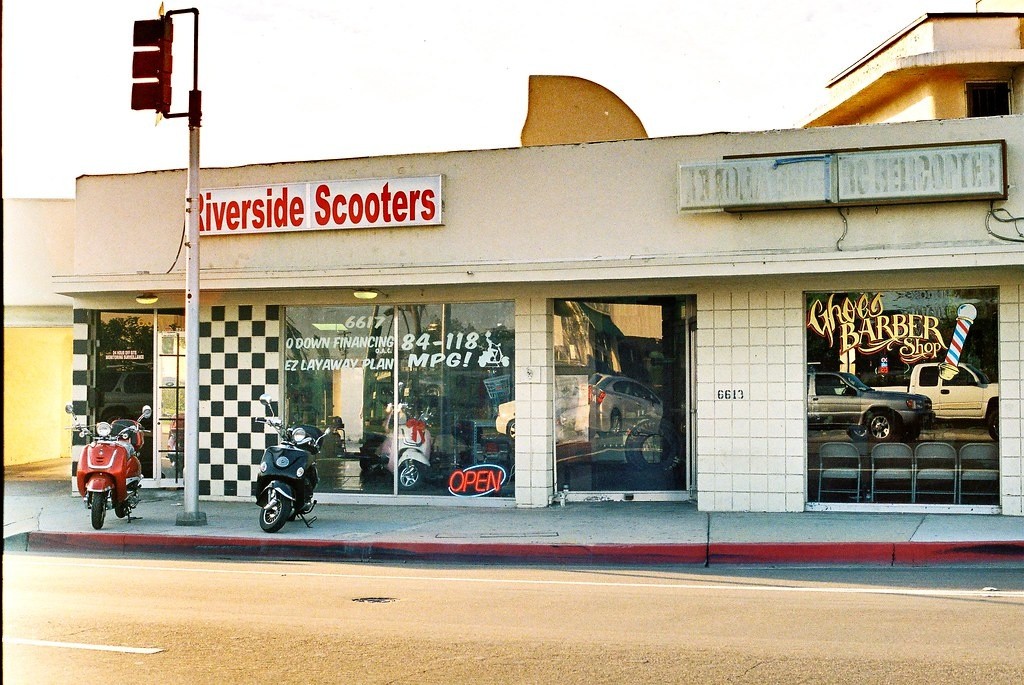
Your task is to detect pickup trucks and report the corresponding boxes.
[846,363,999,442]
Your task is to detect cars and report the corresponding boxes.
[496,400,515,440]
[807,372,935,442]
[588,373,667,433]
[95,369,156,408]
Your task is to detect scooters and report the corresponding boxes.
[255,394,330,532]
[65,405,152,530]
[380,382,433,490]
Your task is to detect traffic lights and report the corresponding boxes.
[131,17,173,110]
